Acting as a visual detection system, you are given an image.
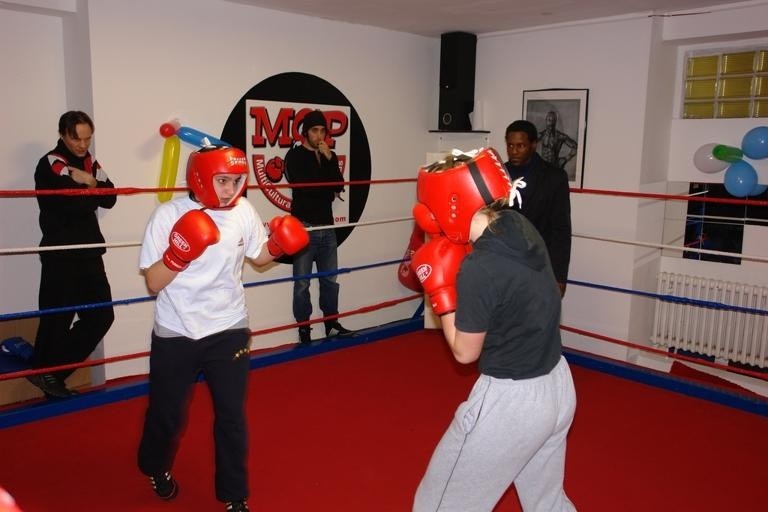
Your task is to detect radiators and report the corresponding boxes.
[650,268,767,369]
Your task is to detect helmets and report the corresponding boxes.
[186,144,248,209]
[417,147,514,243]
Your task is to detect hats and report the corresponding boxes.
[301,112,325,135]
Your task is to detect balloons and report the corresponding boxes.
[724,159,758,197]
[176,126,231,149]
[712,144,743,163]
[157,135,180,203]
[741,126,768,160]
[159,123,175,138]
[693,142,729,174]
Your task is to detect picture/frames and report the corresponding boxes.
[522,87,589,189]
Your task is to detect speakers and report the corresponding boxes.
[438,31,477,131]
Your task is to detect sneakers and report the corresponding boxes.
[299,328,311,344]
[225,500,249,512]
[326,322,352,336]
[150,473,177,499]
[25,372,71,400]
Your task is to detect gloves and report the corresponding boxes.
[267,215,309,256]
[411,239,465,316]
[413,203,444,237]
[163,209,219,272]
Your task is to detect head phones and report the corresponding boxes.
[302,121,327,136]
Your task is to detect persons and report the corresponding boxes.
[286,109,353,345]
[136,143,312,511]
[411,144,579,512]
[536,110,578,168]
[24,109,117,400]
[500,119,571,300]
